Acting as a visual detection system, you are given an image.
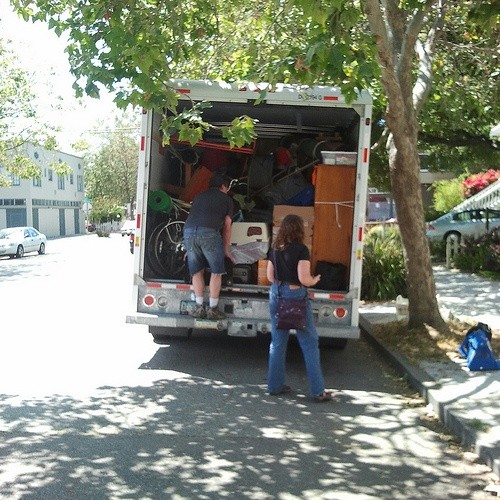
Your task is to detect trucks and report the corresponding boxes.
[124,79,373,351]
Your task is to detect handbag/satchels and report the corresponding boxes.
[314,262,347,290]
[256,167,314,208]
[275,297,307,330]
[459,328,500,372]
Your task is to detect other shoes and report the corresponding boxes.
[271,384,292,396]
[312,391,333,403]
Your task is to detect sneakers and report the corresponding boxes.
[191,304,206,318]
[207,304,226,319]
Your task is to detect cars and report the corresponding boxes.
[0,226,47,258]
[120,220,137,236]
[425,207,500,248]
[85,219,95,232]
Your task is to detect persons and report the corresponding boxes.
[266,214,336,403]
[181,174,236,321]
